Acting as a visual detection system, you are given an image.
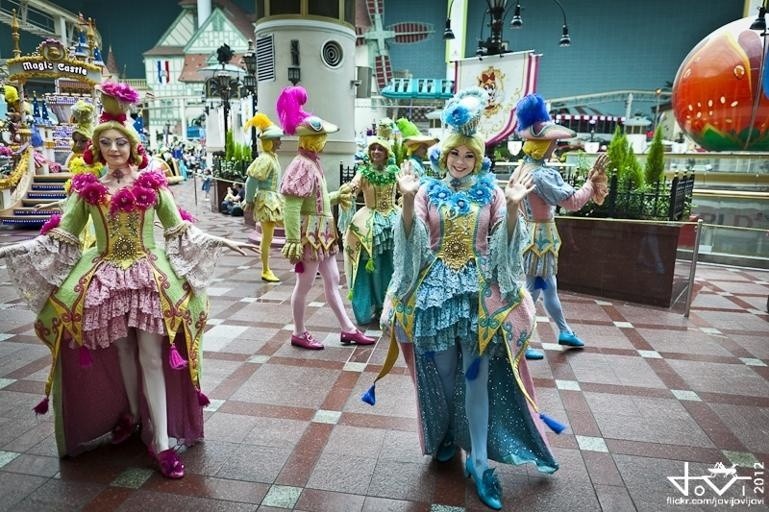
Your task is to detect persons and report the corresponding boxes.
[1,119,259,479]
[244,138,320,282]
[229,183,245,216]
[280,133,377,348]
[34,124,104,251]
[166,135,206,180]
[219,184,238,213]
[362,134,564,510]
[343,138,401,324]
[6,112,22,169]
[506,140,609,359]
[405,144,428,178]
[202,168,212,199]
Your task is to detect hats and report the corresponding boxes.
[71,83,148,169]
[278,86,341,135]
[516,94,577,140]
[367,116,440,162]
[243,111,284,139]
[440,86,486,174]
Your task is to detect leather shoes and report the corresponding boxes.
[341,329,375,344]
[107,411,142,445]
[560,333,583,346]
[147,445,184,479]
[263,272,280,282]
[293,330,325,349]
[437,449,451,461]
[466,457,502,509]
[523,349,543,358]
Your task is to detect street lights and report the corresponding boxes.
[750,6,769,37]
[243,39,257,159]
[218,70,231,152]
[444,0,572,176]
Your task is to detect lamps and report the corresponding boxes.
[444,0,571,62]
